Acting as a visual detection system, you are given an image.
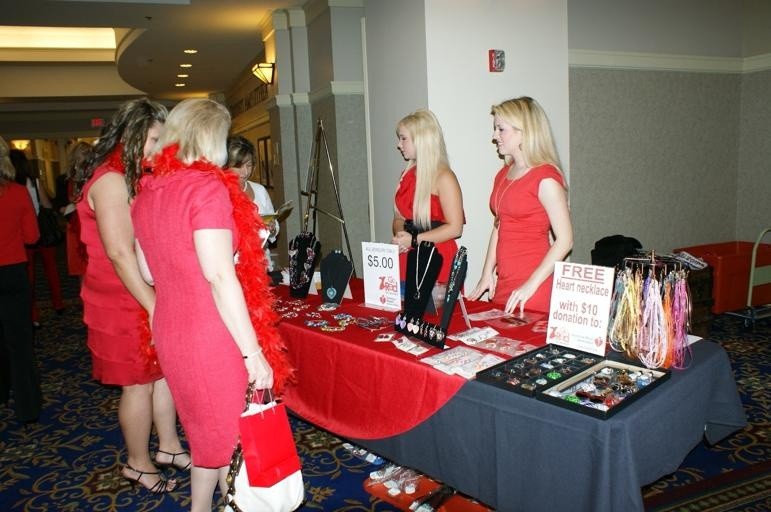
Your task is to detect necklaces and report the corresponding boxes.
[413,245,435,300]
[494,167,528,229]
[447,251,465,302]
[326,254,344,300]
[288,235,317,289]
[242,182,248,192]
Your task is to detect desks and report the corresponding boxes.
[270,276,747,512]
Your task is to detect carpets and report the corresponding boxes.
[364,464,493,512]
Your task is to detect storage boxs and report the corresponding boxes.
[673,241,771,314]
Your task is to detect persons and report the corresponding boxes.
[392,110,466,295]
[0,136,43,424]
[467,96,574,316]
[130,99,274,512]
[72,98,192,496]
[223,136,280,273]
[10,150,66,330]
[53,142,93,282]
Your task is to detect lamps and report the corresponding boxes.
[251,63,274,85]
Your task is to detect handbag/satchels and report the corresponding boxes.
[223,383,305,512]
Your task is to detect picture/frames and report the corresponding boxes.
[257,136,274,187]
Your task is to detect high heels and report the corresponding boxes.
[157,449,191,473]
[123,462,180,494]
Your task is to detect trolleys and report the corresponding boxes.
[722,226,771,329]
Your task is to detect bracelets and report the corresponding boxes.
[412,234,417,248]
[276,300,356,332]
[243,348,263,359]
